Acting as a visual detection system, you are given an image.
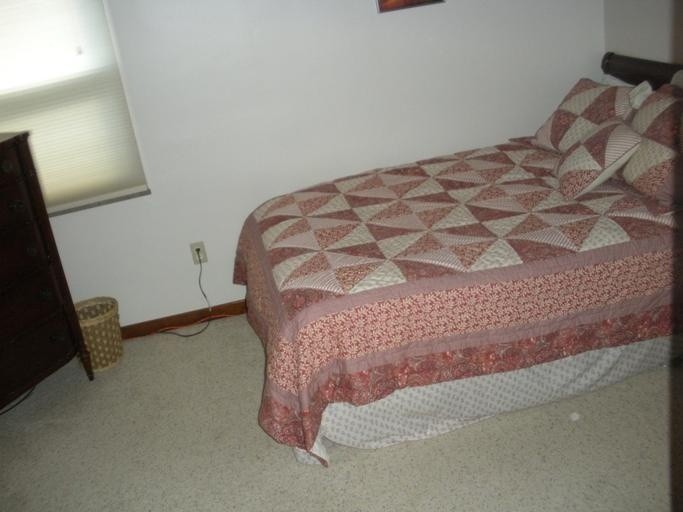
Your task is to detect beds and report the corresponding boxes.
[230,45,681,471]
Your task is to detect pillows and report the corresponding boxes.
[558,115,645,203]
[620,81,681,221]
[528,75,638,154]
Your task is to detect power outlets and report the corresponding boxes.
[190,241,207,264]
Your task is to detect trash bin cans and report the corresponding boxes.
[74,296,125,374]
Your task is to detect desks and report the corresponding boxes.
[0,128,95,411]
